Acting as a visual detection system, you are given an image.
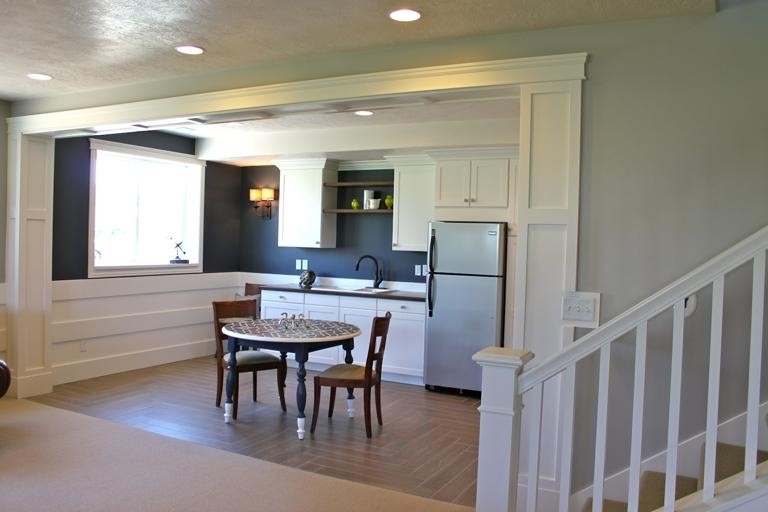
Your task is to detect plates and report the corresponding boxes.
[393,166,439,252]
[436,160,510,221]
[325,181,393,216]
[279,168,337,248]
[260,290,423,385]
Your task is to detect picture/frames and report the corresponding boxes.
[216,318,359,439]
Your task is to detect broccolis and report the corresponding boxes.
[423,221,506,393]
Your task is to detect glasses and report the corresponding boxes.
[247,185,274,220]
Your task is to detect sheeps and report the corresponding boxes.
[355,254,383,289]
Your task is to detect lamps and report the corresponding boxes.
[312,309,391,436]
[213,298,285,414]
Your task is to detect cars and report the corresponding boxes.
[369,199,381,210]
[364,189,374,210]
